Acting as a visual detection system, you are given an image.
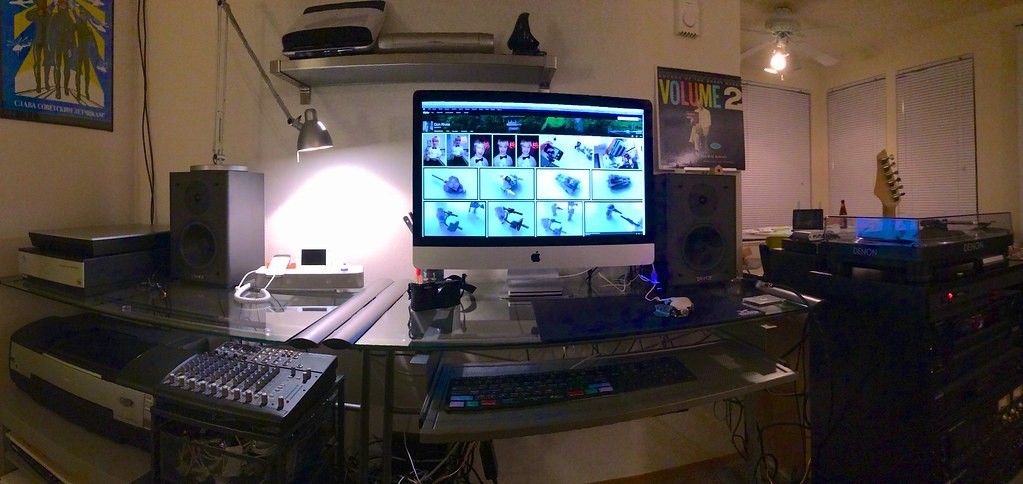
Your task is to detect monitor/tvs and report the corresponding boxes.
[411,89,656,298]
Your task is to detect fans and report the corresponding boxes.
[741,8,840,71]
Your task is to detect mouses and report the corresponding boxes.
[667,297,694,317]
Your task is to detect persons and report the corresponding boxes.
[450,135,464,157]
[493,139,513,165]
[517,139,537,168]
[427,136,441,158]
[689,101,711,151]
[471,138,488,166]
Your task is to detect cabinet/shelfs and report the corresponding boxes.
[0,272,821,484]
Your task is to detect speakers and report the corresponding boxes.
[649,171,735,288]
[169,170,264,287]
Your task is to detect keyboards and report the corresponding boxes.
[445,365,619,411]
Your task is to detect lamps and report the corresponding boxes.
[190,0,334,170]
[770,39,789,71]
